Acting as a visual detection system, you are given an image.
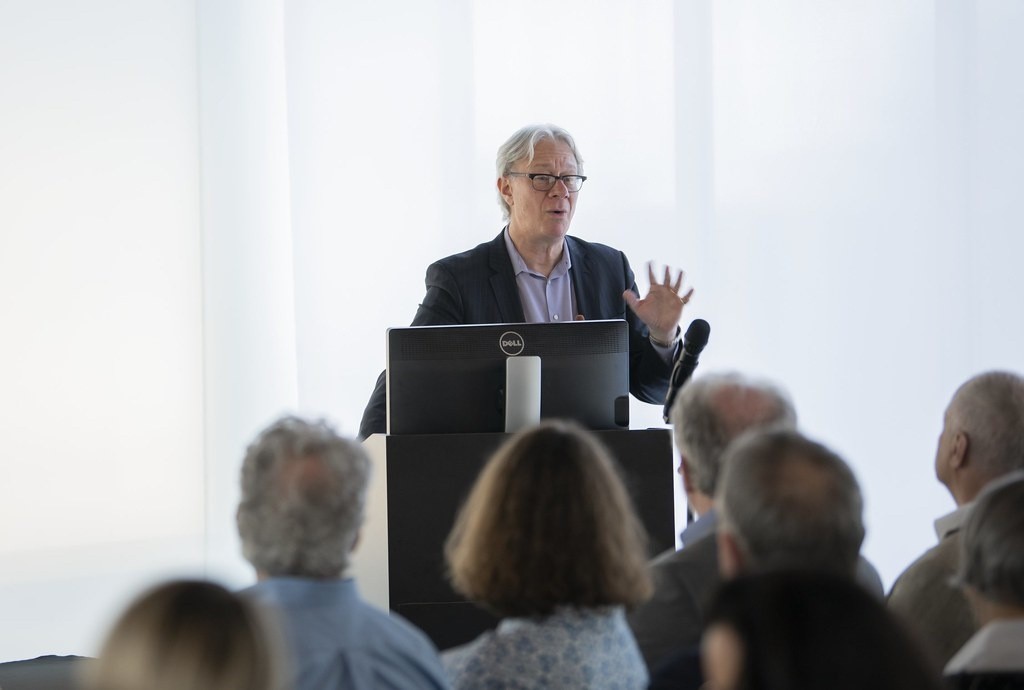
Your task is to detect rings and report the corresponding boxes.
[669,288,677,296]
[679,298,686,305]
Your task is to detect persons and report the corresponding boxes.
[94,370,1024,690]
[355,123,695,445]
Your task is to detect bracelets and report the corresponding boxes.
[650,325,681,350]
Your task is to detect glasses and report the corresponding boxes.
[508,173,586,193]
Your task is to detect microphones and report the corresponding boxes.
[663,318,711,416]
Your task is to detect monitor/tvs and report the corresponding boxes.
[386,319,629,436]
